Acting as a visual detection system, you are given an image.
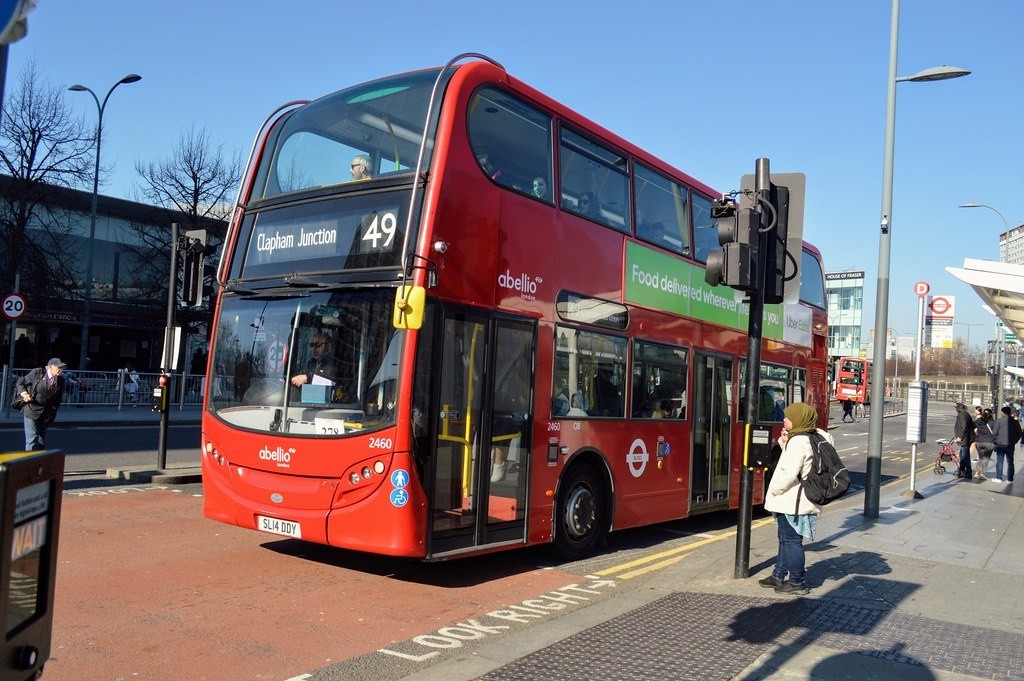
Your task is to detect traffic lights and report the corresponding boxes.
[183,229,217,308]
[704,206,750,289]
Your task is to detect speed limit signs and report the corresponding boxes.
[0,293,27,320]
[267,337,288,374]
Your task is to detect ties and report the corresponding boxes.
[47,376,56,395]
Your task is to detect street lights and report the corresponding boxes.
[68,72,142,394]
[861,0,973,519]
[958,204,1010,419]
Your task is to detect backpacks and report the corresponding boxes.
[785,429,851,505]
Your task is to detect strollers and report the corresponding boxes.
[934,437,964,479]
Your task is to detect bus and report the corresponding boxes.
[200,48,830,563]
[836,358,873,404]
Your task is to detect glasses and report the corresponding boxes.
[309,342,325,348]
[351,164,361,169]
[578,200,589,205]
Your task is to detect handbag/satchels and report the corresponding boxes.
[10,367,46,411]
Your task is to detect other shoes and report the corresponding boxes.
[841,421,845,423]
[131,394,134,396]
[853,420,859,423]
[1020,444,1024,447]
[1007,481,1013,484]
[992,478,1002,483]
[973,458,978,461]
[979,476,987,480]
[132,405,137,407]
[960,474,972,479]
[973,475,978,479]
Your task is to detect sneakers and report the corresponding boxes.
[759,576,783,588]
[508,463,520,473]
[774,582,808,595]
[490,461,507,482]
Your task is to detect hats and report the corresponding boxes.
[48,358,67,367]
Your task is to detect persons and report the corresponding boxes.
[953,403,974,479]
[992,407,1022,484]
[586,359,624,417]
[279,332,342,392]
[1003,399,1024,447]
[553,374,568,417]
[116,363,139,408]
[0,334,61,368]
[349,155,373,180]
[529,173,552,204]
[60,365,84,405]
[632,374,651,416]
[489,367,522,484]
[16,358,65,452]
[974,406,996,480]
[841,396,857,423]
[233,350,252,402]
[759,403,835,595]
[625,206,665,244]
[479,157,503,182]
[760,386,785,421]
[188,347,208,394]
[578,192,609,224]
